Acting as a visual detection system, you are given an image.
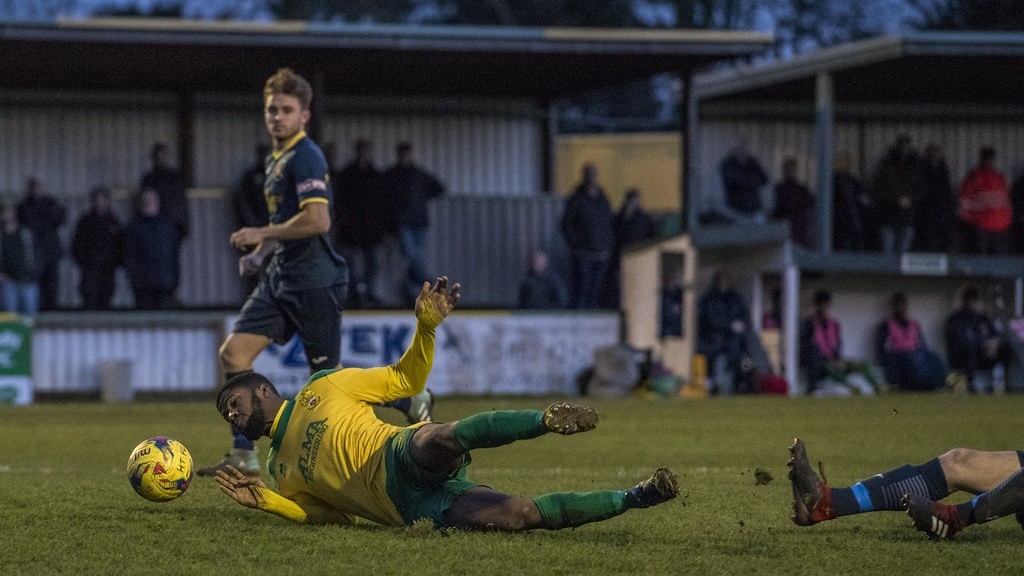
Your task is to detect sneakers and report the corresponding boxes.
[788,438,837,527]
[197,447,260,476]
[542,402,598,434]
[901,493,966,542]
[405,389,434,424]
[632,467,681,508]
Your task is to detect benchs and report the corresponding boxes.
[690,350,1011,396]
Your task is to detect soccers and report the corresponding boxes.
[127,435,195,503]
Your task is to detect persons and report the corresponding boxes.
[799,290,845,393]
[196,70,348,476]
[832,133,1024,257]
[789,439,1024,542]
[0,142,196,312]
[217,277,682,531]
[663,274,682,336]
[518,166,654,310]
[719,137,816,244]
[943,287,1011,395]
[763,288,781,328]
[874,293,935,396]
[698,270,752,394]
[319,136,445,307]
[232,144,270,296]
